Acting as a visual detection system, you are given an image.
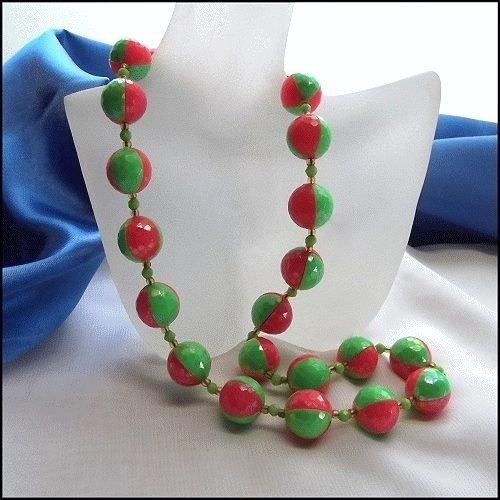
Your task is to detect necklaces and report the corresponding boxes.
[101,39,451,441]
